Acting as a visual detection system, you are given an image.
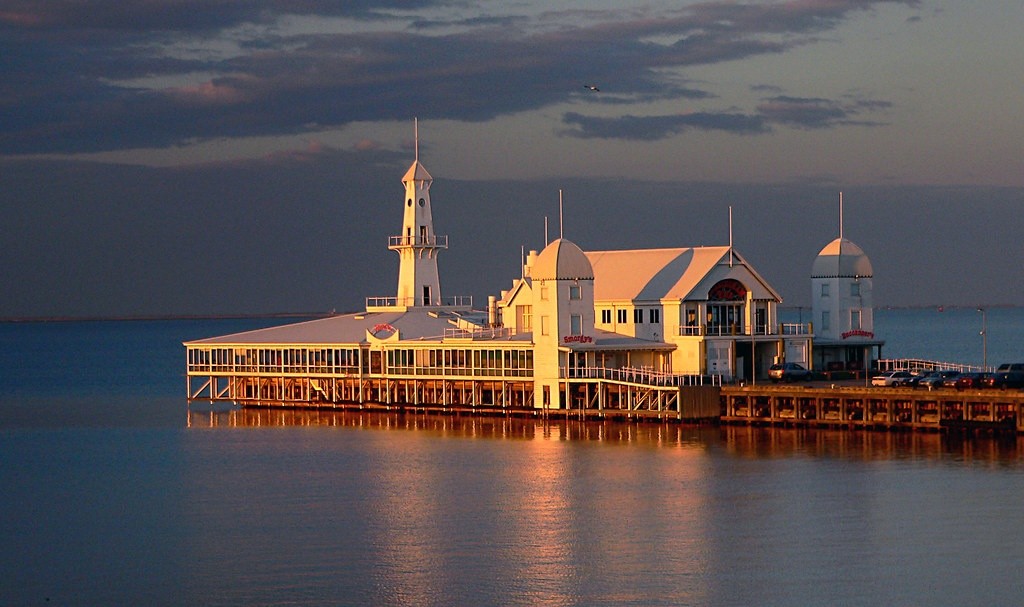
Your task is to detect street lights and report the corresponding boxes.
[977,306,987,378]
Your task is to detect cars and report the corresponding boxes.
[870,363,1024,390]
[768,362,815,383]
